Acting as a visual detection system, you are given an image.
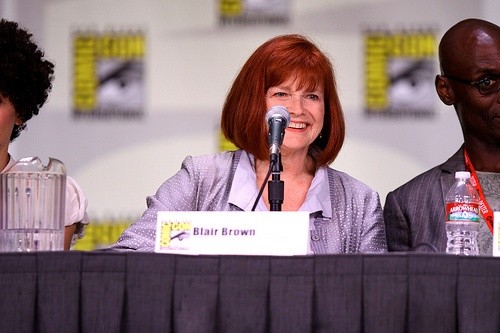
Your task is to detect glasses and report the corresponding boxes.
[444,75,500,95]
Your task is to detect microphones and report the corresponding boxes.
[265,105,291,162]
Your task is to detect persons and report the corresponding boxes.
[383,19,500,253]
[106,34,388,254]
[0,19,90,251]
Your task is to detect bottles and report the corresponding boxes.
[444,171,481,255]
